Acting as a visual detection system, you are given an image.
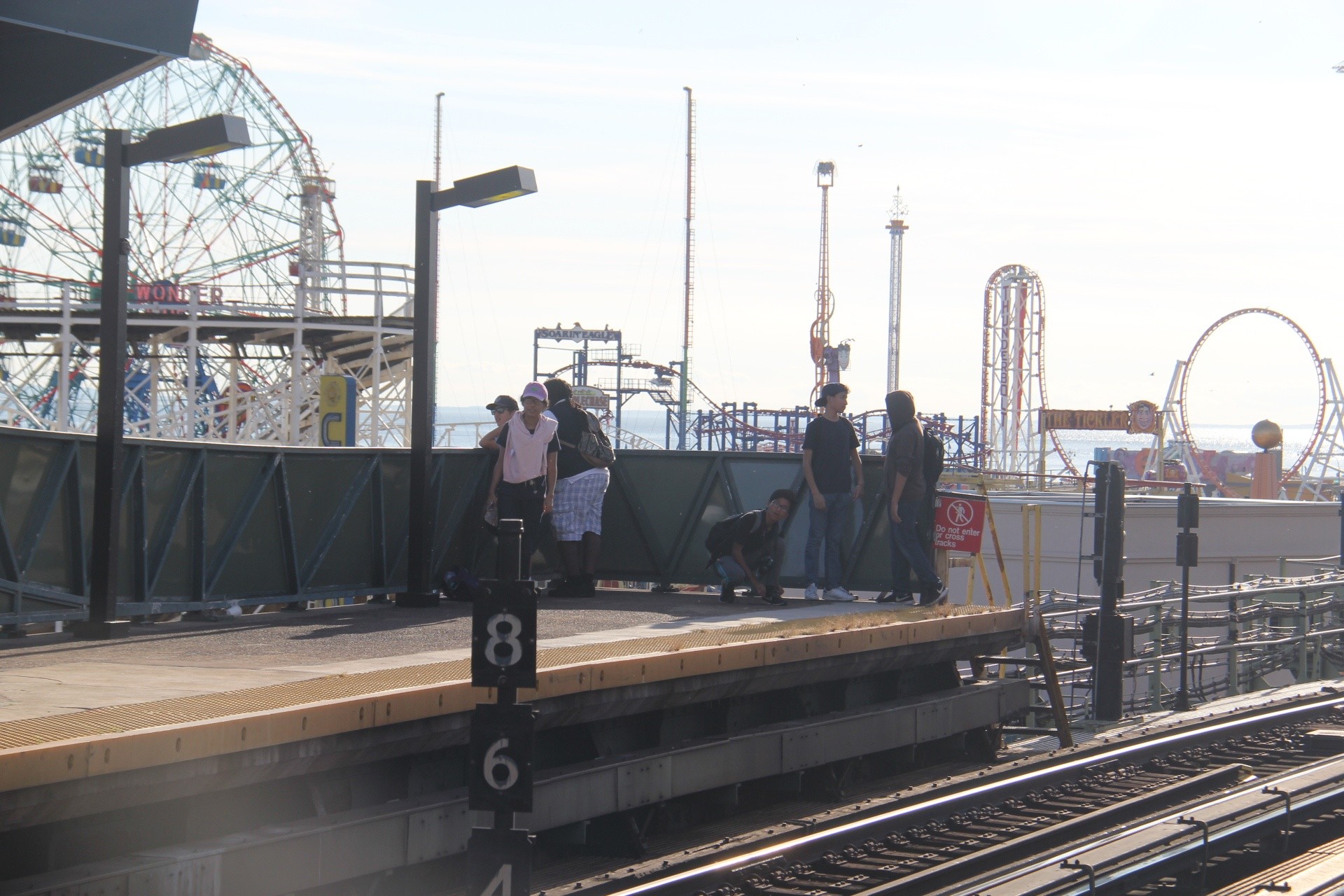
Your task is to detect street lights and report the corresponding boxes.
[403,165,539,608]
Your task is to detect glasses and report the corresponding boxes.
[772,499,791,513]
[524,398,545,405]
[491,407,509,415]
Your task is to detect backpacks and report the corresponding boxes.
[923,426,946,483]
[559,408,616,469]
[704,510,763,571]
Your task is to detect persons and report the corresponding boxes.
[712,488,794,606]
[542,379,610,599]
[802,382,865,601]
[877,390,949,607]
[478,395,519,451]
[486,382,562,580]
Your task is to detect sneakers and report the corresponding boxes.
[925,579,949,606]
[719,585,736,604]
[822,586,859,601]
[879,592,916,605]
[761,590,788,606]
[804,582,820,601]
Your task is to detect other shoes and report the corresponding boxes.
[548,574,596,598]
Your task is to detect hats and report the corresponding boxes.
[486,395,519,411]
[522,381,549,401]
[814,382,847,407]
